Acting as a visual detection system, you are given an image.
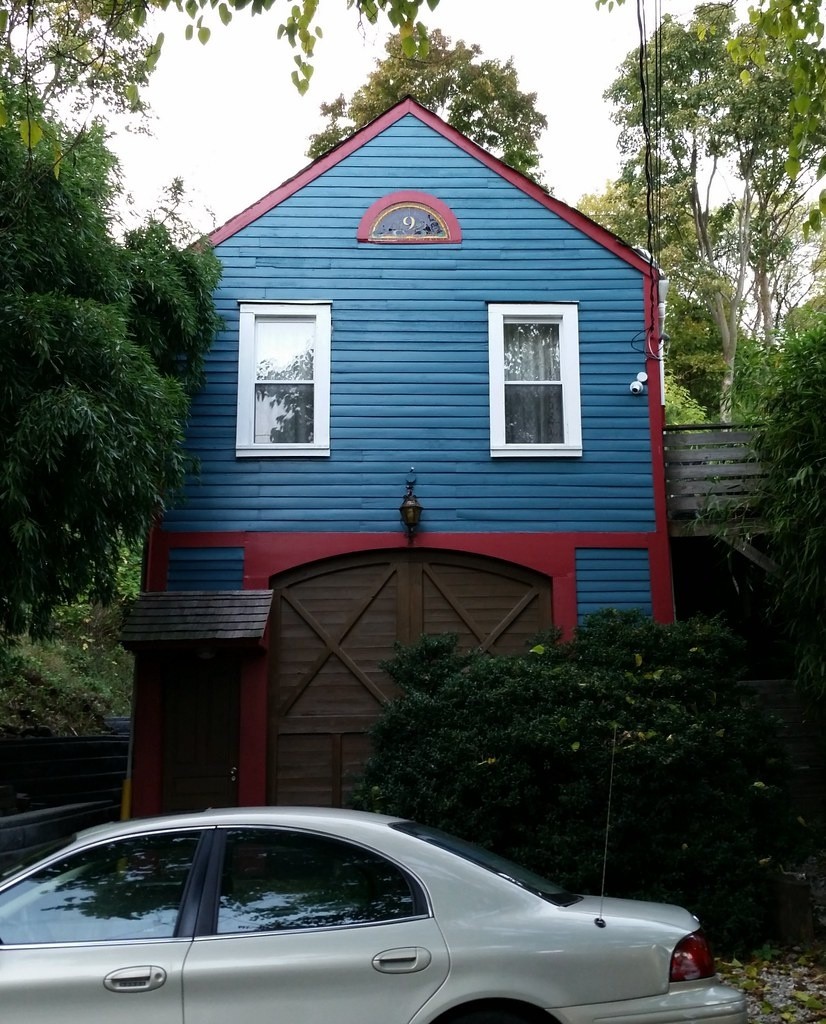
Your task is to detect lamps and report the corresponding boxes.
[399,485,422,542]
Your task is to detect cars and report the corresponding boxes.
[0,808,750,1023]
